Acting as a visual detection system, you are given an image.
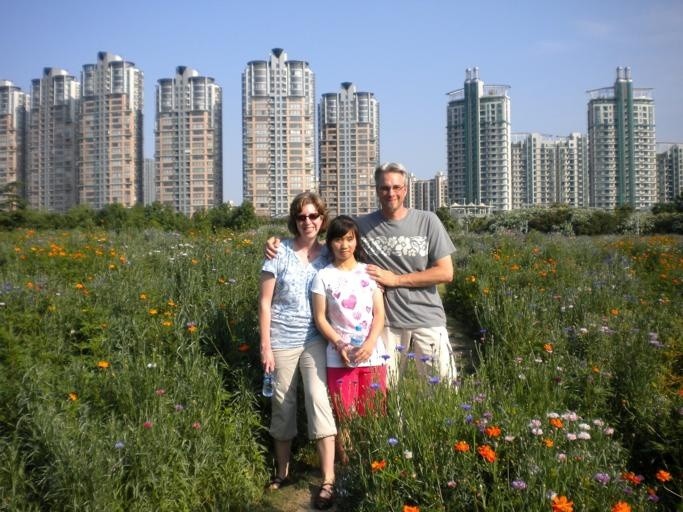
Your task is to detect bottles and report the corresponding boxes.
[262,367,275,398]
[346,326,364,369]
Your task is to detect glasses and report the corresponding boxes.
[377,186,404,193]
[296,213,320,222]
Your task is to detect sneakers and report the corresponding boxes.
[313,481,336,510]
[266,475,288,490]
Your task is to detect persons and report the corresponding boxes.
[256,190,338,510]
[308,214,389,472]
[263,161,458,426]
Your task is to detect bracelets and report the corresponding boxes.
[334,338,346,353]
